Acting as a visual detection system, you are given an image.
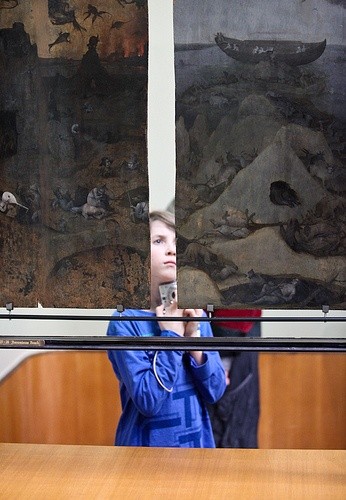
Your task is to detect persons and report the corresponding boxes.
[107,210,229,447]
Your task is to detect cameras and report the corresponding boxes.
[159,282,178,311]
